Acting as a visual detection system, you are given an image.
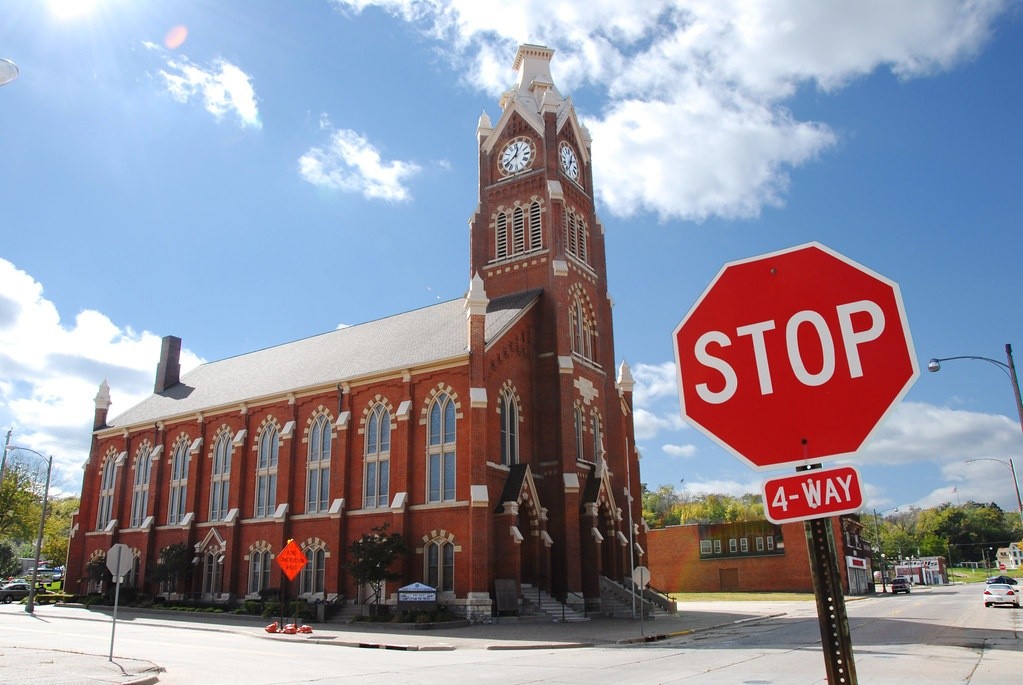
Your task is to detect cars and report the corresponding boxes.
[983,584,1019,608]
[986,575,1018,585]
[0,583,56,604]
[9,579,26,583]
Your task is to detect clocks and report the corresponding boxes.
[561,147,578,178]
[501,141,531,173]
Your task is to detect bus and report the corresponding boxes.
[23,568,63,594]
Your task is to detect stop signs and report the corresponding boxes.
[673,241,922,470]
[999,564,1006,571]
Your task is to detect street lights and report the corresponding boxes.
[5,445,52,612]
[929,357,1021,439]
[947,542,954,583]
[982,547,993,576]
[964,458,1022,521]
[874,507,899,593]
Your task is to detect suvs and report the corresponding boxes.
[892,577,910,594]
